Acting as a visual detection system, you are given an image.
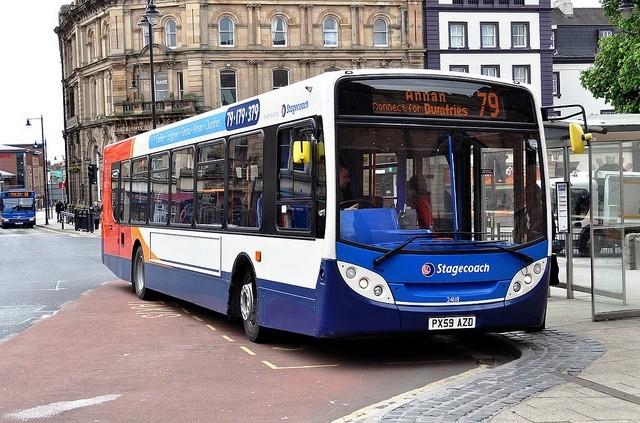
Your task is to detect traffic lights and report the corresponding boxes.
[88,163,97,184]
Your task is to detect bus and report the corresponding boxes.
[101,68,593,342]
[0,189,35,228]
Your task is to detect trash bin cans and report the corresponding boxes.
[74,208,96,233]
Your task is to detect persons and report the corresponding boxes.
[336,161,358,210]
[55,199,63,223]
[93,201,102,230]
[407,173,433,229]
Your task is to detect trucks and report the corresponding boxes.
[535,170,640,255]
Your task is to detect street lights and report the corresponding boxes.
[26,115,49,224]
[142,0,162,128]
[54,155,64,200]
[33,139,50,219]
[552,149,560,177]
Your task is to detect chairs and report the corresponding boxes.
[258,193,289,229]
[181,203,195,223]
